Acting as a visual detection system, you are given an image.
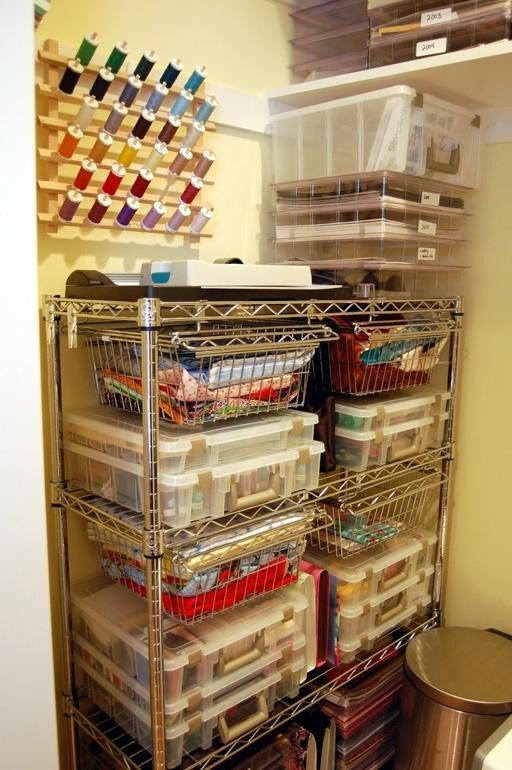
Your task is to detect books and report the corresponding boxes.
[280,628,408,769]
[275,5,510,269]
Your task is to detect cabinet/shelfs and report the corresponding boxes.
[43,283,464,770]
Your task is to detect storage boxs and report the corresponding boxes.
[260,74,496,196]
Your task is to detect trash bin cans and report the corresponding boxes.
[395,627,512,770]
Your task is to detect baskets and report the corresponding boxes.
[307,473,449,559]
[76,344,338,426]
[310,333,463,396]
[95,526,305,626]
